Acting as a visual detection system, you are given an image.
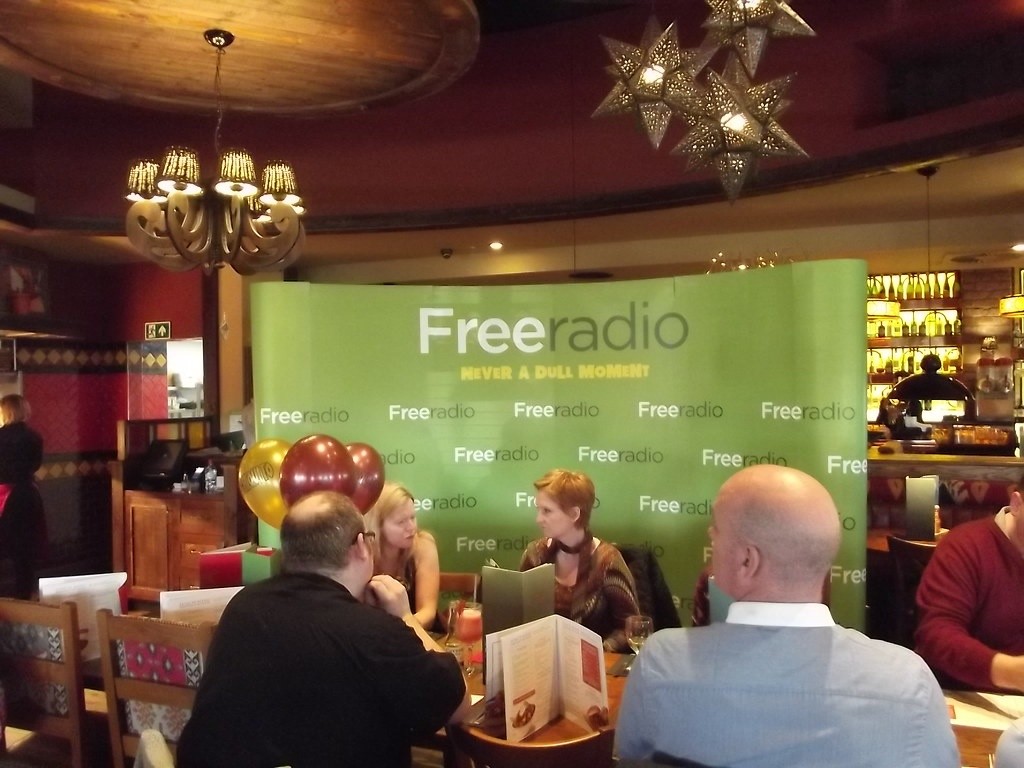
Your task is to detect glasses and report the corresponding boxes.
[353,530,375,545]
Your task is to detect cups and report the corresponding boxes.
[625,616,654,655]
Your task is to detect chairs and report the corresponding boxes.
[888,535,941,640]
[438,544,680,768]
[447,720,614,768]
[0,597,218,768]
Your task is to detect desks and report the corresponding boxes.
[448,650,1024,768]
[79,604,159,693]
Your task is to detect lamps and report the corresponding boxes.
[981,335,998,352]
[888,164,1024,405]
[589,0,816,204]
[124,30,306,277]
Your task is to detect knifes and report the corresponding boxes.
[614,654,635,676]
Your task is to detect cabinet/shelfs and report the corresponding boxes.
[124,490,224,601]
[868,268,962,423]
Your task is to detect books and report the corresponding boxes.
[906,474,939,541]
[159,586,246,624]
[481,558,608,744]
[199,542,283,588]
[39,572,128,664]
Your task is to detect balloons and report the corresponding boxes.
[238,433,386,532]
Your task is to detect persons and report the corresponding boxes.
[0,393,46,601]
[914,467,1024,694]
[613,463,958,768]
[358,483,446,634]
[520,468,641,652]
[175,492,472,768]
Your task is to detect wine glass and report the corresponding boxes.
[454,602,482,679]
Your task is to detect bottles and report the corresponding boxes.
[181,474,191,495]
[902,322,909,337]
[919,322,926,336]
[204,460,217,495]
[870,362,875,373]
[867,270,960,298]
[911,322,917,336]
[935,505,940,533]
[952,424,999,444]
[945,321,952,336]
[954,316,962,335]
[878,321,885,337]
[445,601,465,668]
